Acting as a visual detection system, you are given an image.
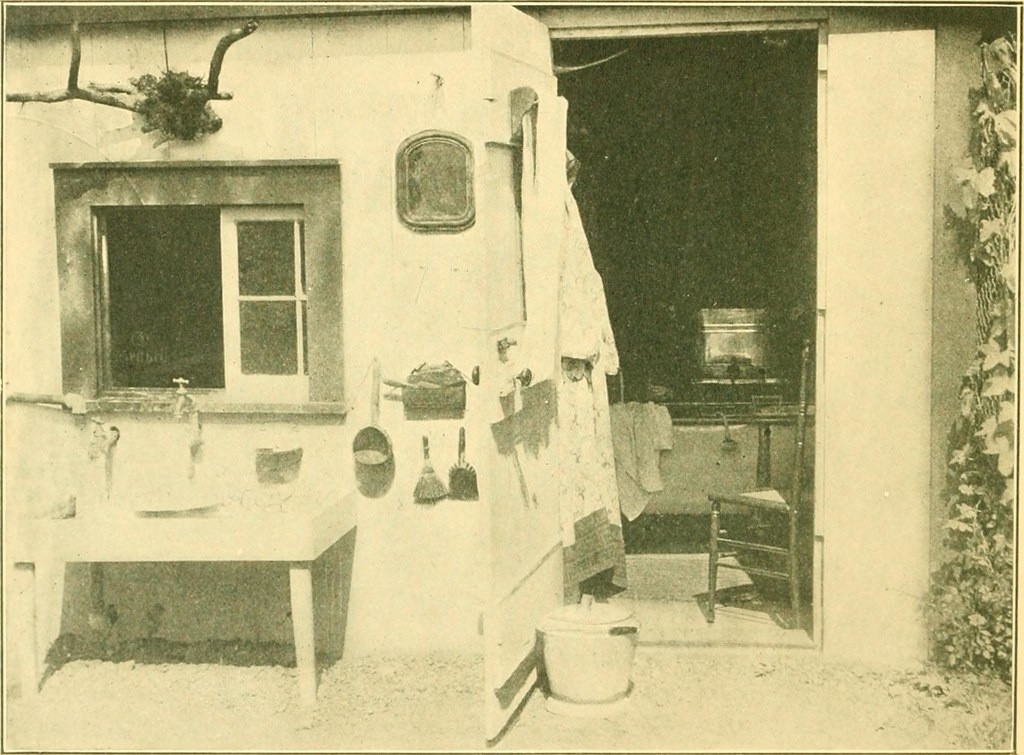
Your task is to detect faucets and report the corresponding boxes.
[170,378,198,423]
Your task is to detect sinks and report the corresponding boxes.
[14,484,357,561]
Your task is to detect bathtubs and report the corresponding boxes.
[615,400,815,514]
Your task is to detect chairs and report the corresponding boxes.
[706,340,814,627]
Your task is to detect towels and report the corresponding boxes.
[609,401,674,522]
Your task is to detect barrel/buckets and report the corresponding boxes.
[539,592,641,703]
